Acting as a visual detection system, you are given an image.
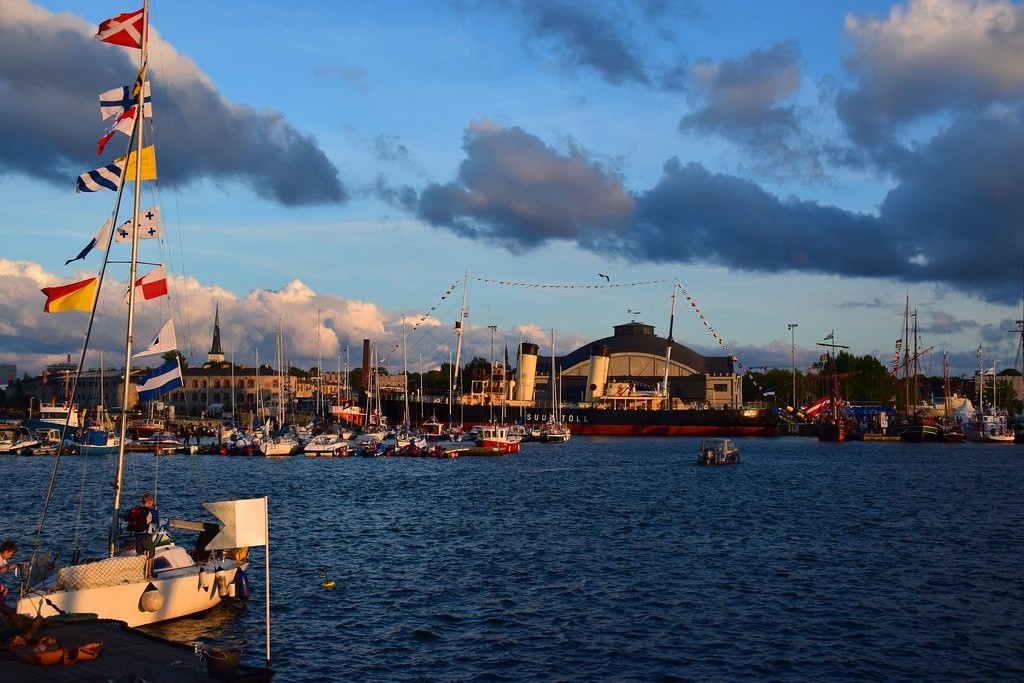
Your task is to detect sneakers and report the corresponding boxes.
[147,572,156,581]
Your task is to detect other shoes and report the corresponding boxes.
[1,604,16,615]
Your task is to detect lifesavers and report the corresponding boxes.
[114,440,118,445]
[703,404,708,410]
[63,404,68,411]
[72,404,76,411]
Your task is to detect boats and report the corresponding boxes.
[695,438,740,466]
[0,605,276,683]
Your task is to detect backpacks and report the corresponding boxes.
[127,507,149,532]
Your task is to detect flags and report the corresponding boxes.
[75,59,156,194]
[131,318,177,358]
[114,206,162,243]
[123,262,172,307]
[135,355,188,401]
[65,216,114,267]
[39,275,98,313]
[94,7,149,50]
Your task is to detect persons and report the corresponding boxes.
[0,540,25,607]
[120,494,160,578]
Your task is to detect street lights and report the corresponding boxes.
[788,324,799,408]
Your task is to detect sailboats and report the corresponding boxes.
[778,288,1024,443]
[0,0,252,628]
[0,293,572,458]
